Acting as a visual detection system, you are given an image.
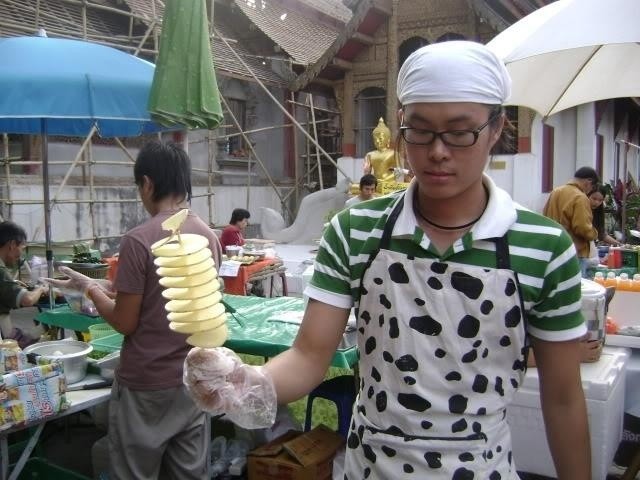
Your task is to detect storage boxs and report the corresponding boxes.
[504,346,630,480]
[6,419,108,480]
[246,423,346,480]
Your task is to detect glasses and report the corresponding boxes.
[396,110,501,149]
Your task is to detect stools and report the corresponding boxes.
[305,375,361,438]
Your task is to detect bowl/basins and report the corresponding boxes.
[243,253,266,261]
[226,249,239,258]
[23,339,92,384]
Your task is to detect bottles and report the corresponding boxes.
[593,245,640,292]
[607,320,616,334]
[0,338,23,377]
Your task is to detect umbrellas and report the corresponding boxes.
[0,33,193,339]
[475,0,639,122]
[142,0,228,146]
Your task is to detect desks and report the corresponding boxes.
[585,245,639,351]
[58,260,110,281]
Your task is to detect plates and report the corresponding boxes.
[55,259,110,270]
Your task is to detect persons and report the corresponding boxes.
[585,185,624,247]
[361,116,405,185]
[46,136,227,480]
[216,208,268,299]
[524,332,603,368]
[1,220,53,336]
[182,37,595,480]
[541,165,600,281]
[343,174,379,210]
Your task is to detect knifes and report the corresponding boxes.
[65,382,114,391]
